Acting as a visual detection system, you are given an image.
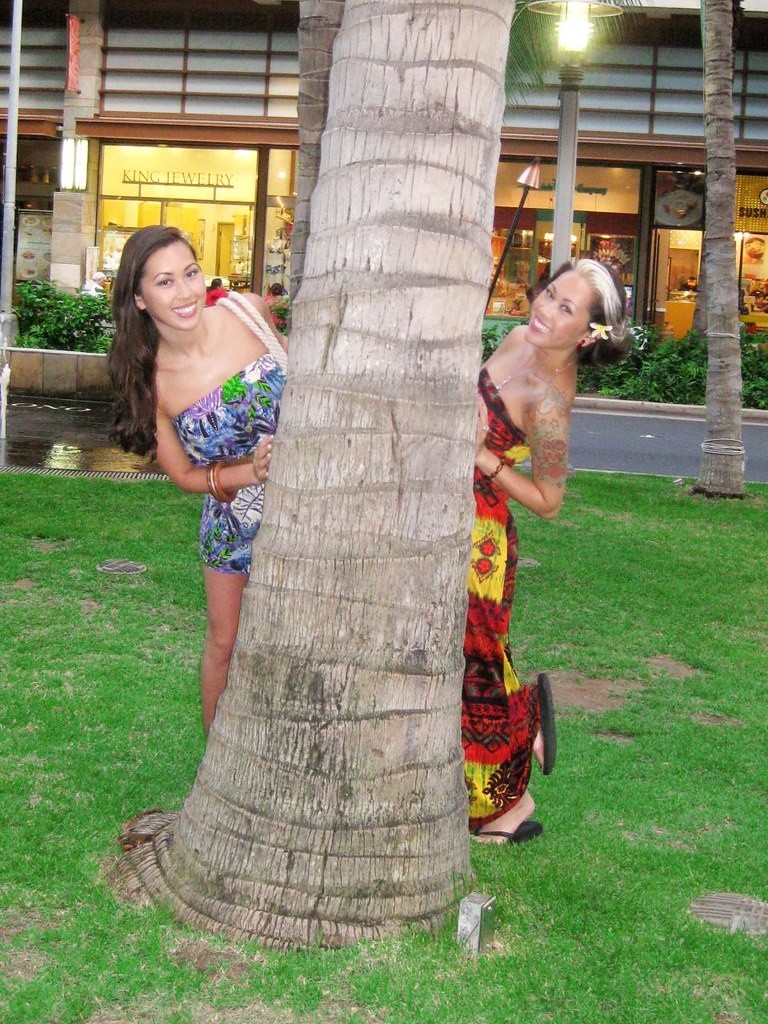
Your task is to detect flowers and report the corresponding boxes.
[590,323,613,340]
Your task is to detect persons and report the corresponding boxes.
[206,278,229,306]
[85,273,107,297]
[463,258,633,843]
[265,283,283,302]
[103,226,290,741]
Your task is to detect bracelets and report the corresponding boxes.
[207,461,237,501]
[487,460,505,480]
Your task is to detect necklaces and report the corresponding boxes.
[496,349,573,389]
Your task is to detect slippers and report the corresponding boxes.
[471,821,542,846]
[533,673,556,777]
